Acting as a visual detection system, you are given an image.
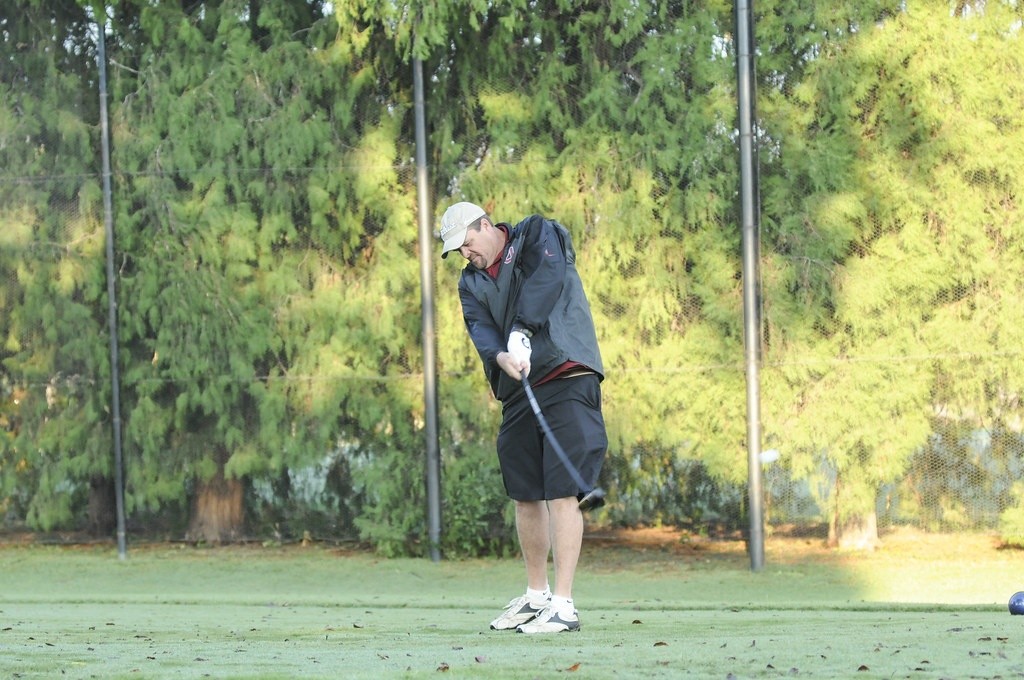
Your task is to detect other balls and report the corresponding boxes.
[1008,591,1024,615]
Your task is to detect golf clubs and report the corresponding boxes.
[519,367,607,513]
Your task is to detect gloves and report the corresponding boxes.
[507,331,532,378]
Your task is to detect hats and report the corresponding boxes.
[440,202,486,259]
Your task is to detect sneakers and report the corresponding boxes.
[516,606,581,635]
[490,593,553,630]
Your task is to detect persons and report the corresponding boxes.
[441,202,605,634]
